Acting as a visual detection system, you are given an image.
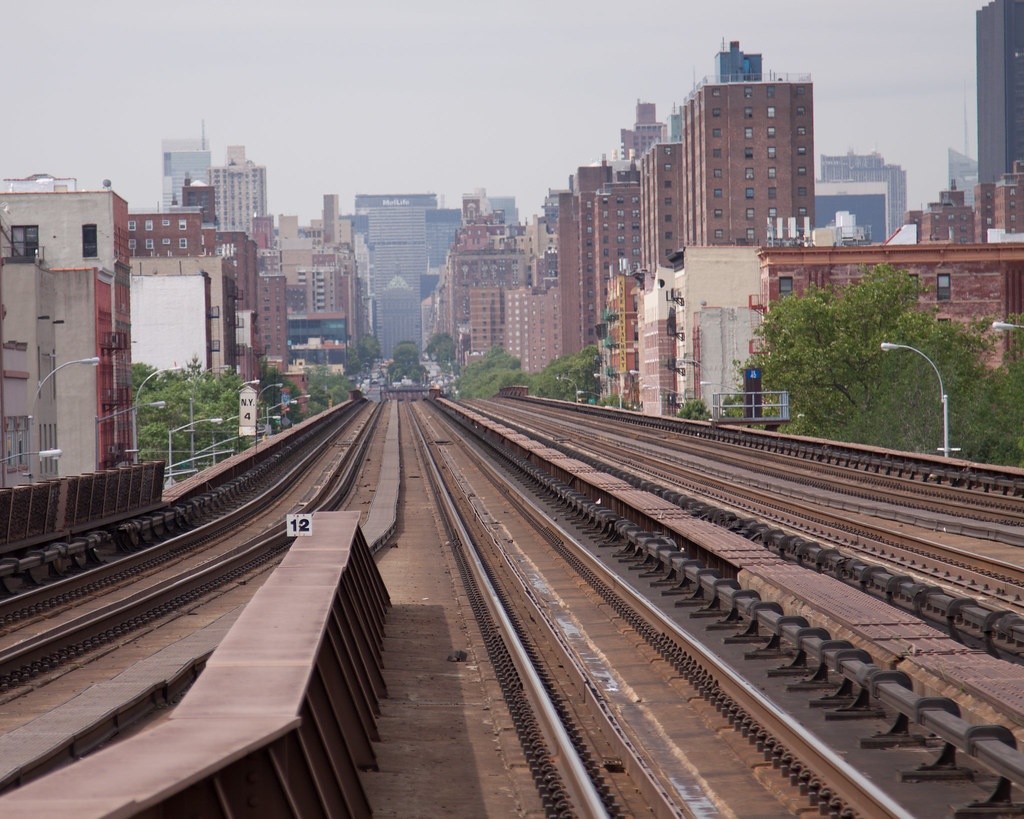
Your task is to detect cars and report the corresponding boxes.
[371,378,377,384]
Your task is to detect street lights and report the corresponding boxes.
[189,364,232,472]
[629,369,663,416]
[592,373,622,410]
[880,340,949,458]
[677,358,717,393]
[132,366,183,464]
[266,394,312,435]
[168,417,223,484]
[94,401,166,472]
[554,376,579,404]
[28,356,101,482]
